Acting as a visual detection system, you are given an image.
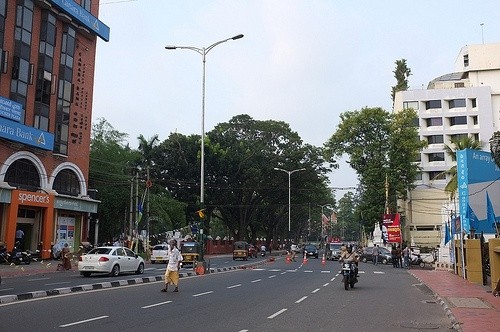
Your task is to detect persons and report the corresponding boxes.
[99,236,130,248]
[290,243,298,262]
[372,244,379,265]
[338,244,358,283]
[159,240,183,292]
[391,245,409,269]
[15,228,24,248]
[61,243,72,270]
[261,245,267,254]
[339,243,346,254]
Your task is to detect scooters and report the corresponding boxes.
[337,255,360,290]
[0,241,32,265]
[260,251,265,257]
[405,253,426,268]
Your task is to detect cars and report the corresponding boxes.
[248,244,259,258]
[302,244,319,259]
[360,246,391,264]
[78,245,145,278]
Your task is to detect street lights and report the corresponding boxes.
[337,215,345,240]
[164,33,246,273]
[381,154,391,218]
[273,167,307,236]
[317,204,332,248]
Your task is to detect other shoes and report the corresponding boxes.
[173,289,178,292]
[161,289,167,292]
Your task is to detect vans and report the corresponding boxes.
[326,242,345,261]
[151,243,172,264]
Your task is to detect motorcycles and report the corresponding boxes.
[232,241,249,261]
[179,241,202,268]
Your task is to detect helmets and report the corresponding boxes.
[346,244,352,254]
